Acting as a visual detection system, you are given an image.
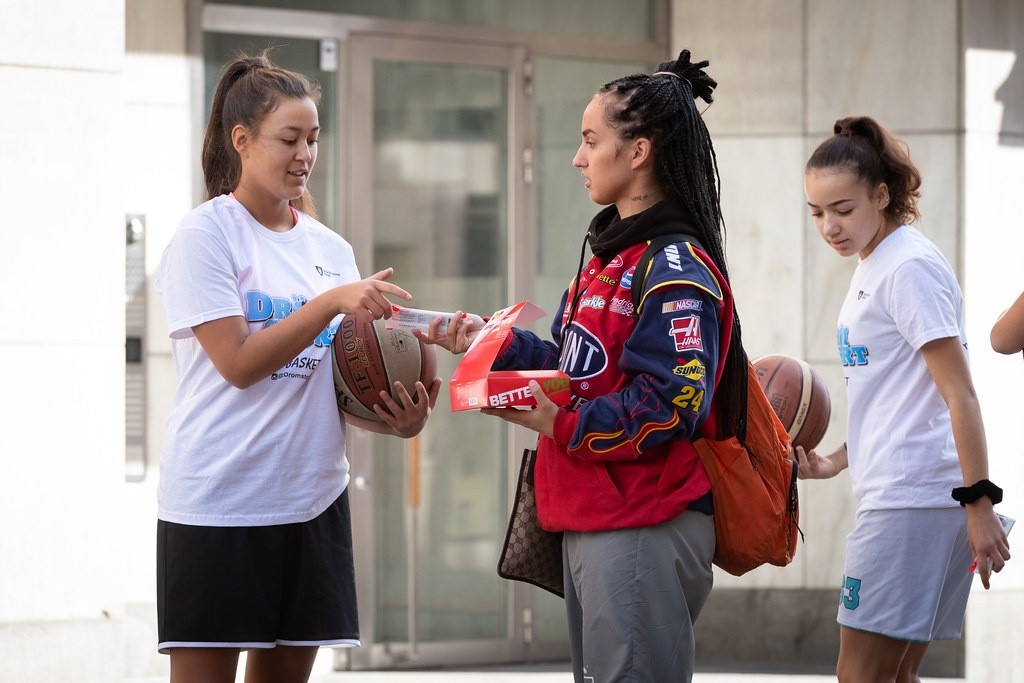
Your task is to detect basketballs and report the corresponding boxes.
[332,302,438,420]
[750,352,831,459]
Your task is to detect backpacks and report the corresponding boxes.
[625,231,804,577]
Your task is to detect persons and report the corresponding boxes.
[155,57,442,683]
[787,117,1024,683]
[411,50,734,683]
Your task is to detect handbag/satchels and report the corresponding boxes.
[492,444,572,596]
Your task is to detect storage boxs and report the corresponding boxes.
[450,299,572,412]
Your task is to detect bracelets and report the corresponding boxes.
[951,479,1003,508]
